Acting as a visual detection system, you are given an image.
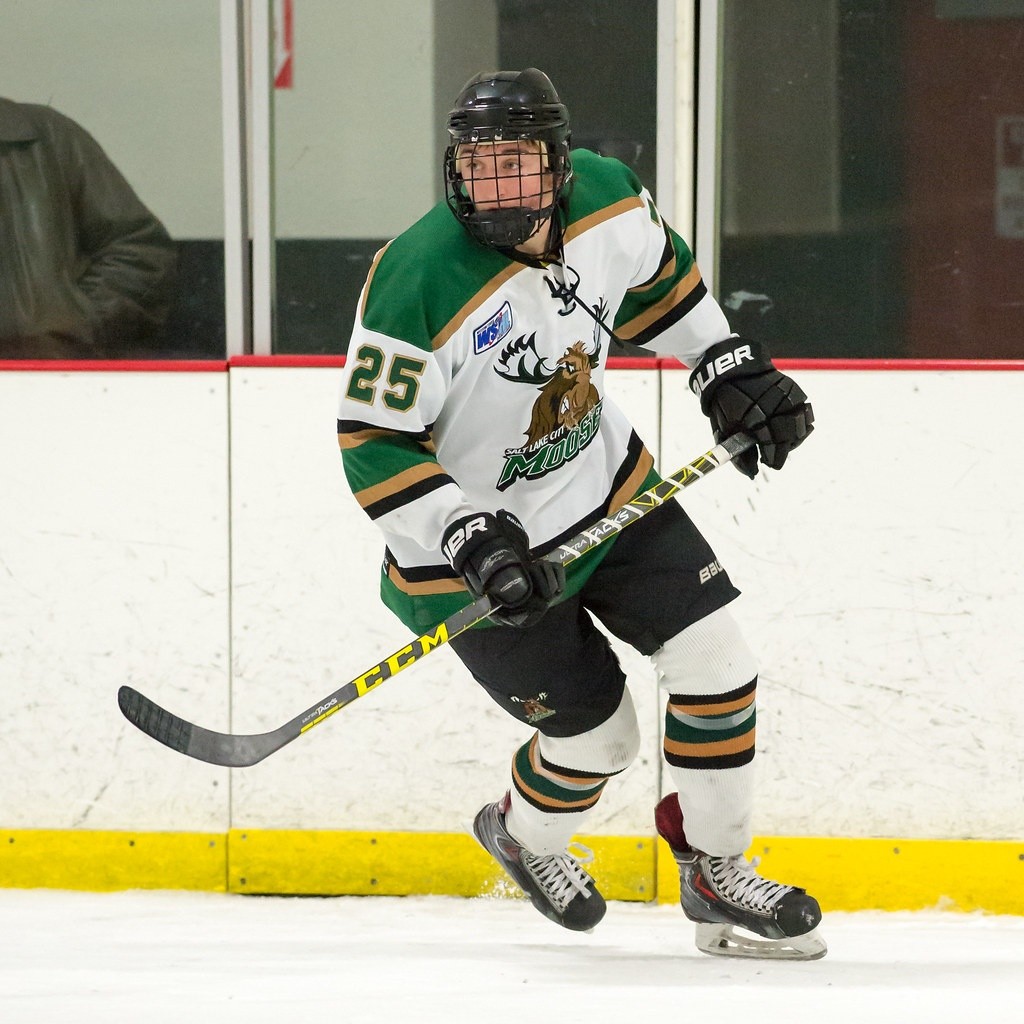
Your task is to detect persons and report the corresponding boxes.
[335,67,827,961]
[1,99,186,360]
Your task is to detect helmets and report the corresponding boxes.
[443,68,573,174]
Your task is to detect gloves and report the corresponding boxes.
[440,512,566,631]
[687,337,816,479]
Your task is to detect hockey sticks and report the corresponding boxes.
[118,427,754,769]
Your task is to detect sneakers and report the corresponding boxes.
[655,793,825,962]
[472,787,607,935]
